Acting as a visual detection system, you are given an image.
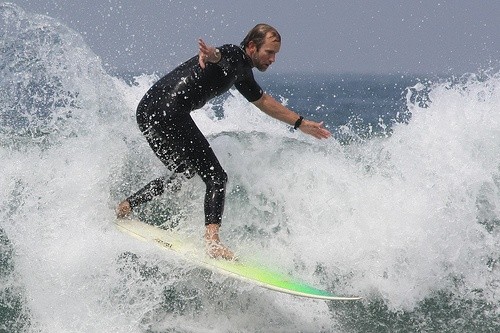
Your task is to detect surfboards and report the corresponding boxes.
[109,218,362,301]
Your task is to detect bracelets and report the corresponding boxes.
[293,116,306,131]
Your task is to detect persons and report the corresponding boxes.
[115,23,332,264]
